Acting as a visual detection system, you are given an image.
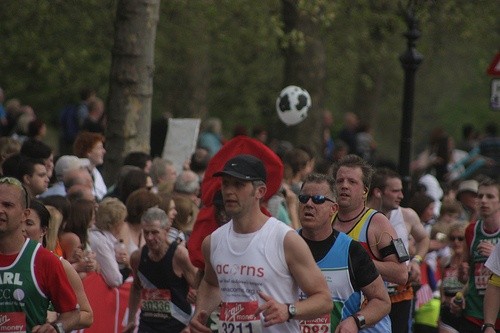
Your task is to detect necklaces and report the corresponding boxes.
[337,206,365,222]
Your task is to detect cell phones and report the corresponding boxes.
[392,238,409,263]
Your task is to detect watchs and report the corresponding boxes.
[481,322,496,333]
[286,303,296,323]
[352,313,366,332]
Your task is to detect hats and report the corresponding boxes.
[455,180,482,200]
[211,154,267,183]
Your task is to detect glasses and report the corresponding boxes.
[0,176,29,209]
[297,194,335,205]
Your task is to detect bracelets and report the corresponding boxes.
[412,254,423,265]
[50,321,65,333]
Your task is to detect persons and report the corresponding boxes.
[330,154,410,333]
[0,85,500,333]
[295,173,391,333]
[190,154,334,333]
